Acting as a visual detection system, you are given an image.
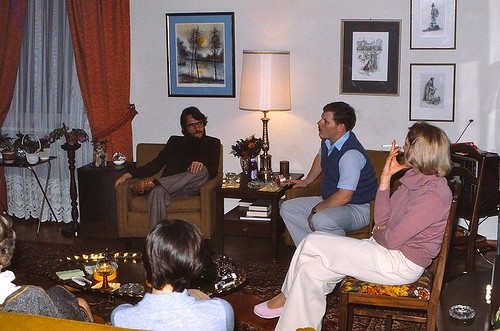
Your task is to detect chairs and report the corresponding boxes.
[338,143,485,331]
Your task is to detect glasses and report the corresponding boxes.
[185,121,203,130]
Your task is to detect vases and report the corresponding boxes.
[240,156,251,175]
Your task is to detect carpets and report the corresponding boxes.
[11,241,439,331]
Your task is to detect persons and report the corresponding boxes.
[253,121,452,331]
[111,218,234,331]
[280,102,378,248]
[0,212,94,323]
[114,107,220,230]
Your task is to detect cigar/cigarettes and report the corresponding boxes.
[383,145,398,147]
[290,177,292,184]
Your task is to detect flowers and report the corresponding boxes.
[230,134,263,161]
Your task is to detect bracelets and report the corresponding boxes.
[311,210,316,214]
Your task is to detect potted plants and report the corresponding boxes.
[0,133,51,164]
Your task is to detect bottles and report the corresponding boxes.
[248,154,258,182]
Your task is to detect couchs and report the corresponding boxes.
[284,151,404,247]
[116,143,224,240]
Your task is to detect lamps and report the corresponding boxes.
[239,51,292,180]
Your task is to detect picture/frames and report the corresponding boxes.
[165,11,235,97]
[338,19,402,97]
[409,63,456,122]
[410,0,457,50]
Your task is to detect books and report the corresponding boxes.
[238,199,271,221]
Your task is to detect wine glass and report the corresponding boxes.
[95,260,114,291]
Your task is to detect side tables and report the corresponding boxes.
[76,160,136,241]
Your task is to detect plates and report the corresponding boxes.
[247,180,264,189]
[119,283,145,295]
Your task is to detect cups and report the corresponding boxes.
[213,254,234,277]
[268,174,278,190]
[280,161,289,175]
[226,173,236,186]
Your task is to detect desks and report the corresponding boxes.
[0,156,58,235]
[436,272,500,331]
[47,250,249,302]
[213,171,303,258]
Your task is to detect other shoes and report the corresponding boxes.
[131,176,155,196]
[253,300,284,319]
